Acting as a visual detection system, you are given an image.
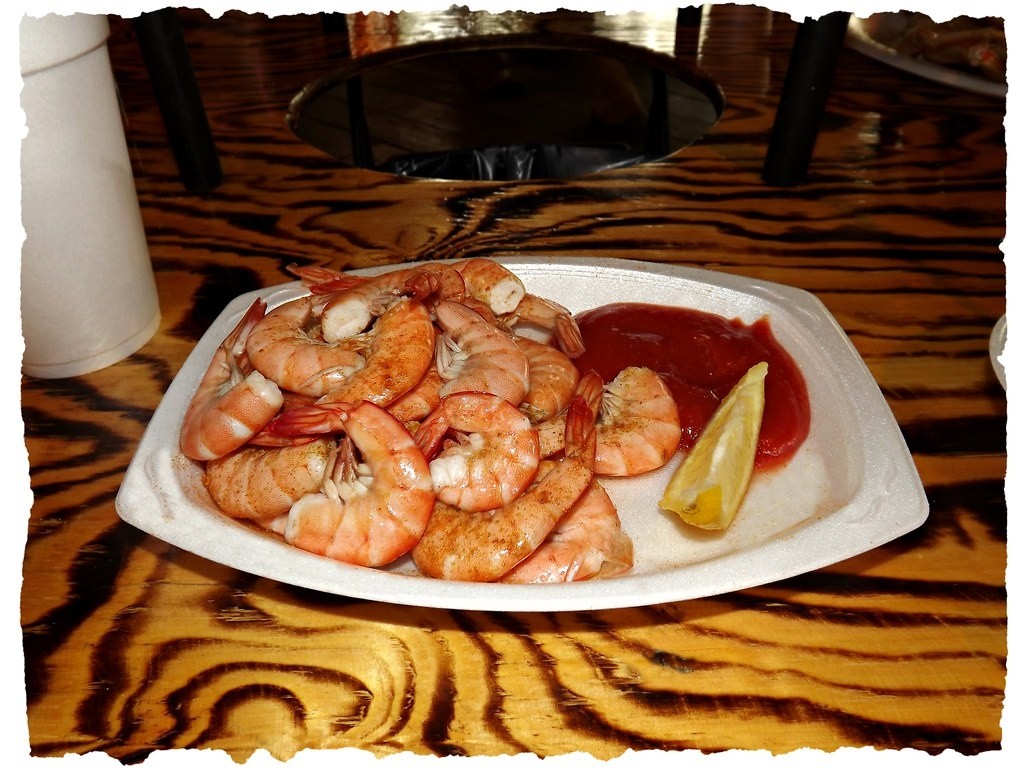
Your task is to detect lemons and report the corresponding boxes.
[659,361,769,529]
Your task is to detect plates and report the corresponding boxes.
[113,252,926,614]
[987,313,1008,391]
[843,17,1008,102]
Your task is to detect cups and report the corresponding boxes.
[22,12,163,378]
